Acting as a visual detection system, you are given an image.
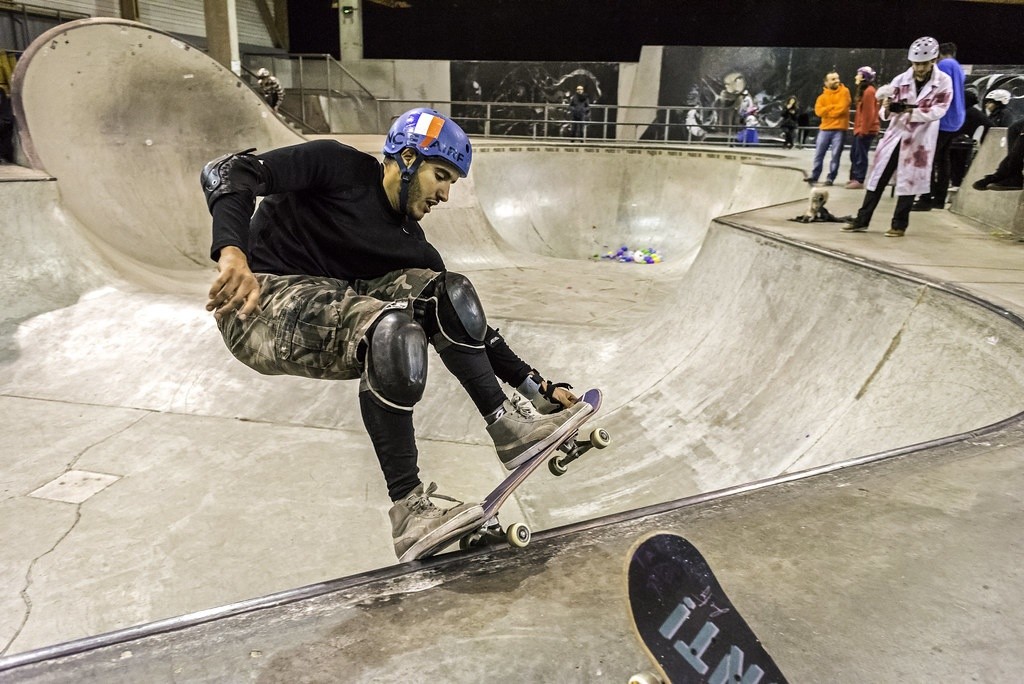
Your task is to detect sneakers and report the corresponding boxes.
[485,393,593,470]
[389,482,484,564]
[987,175,1022,190]
[973,173,1006,190]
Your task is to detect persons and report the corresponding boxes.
[803,71,852,186]
[780,95,800,149]
[202,108,593,561]
[569,85,591,143]
[840,36,954,237]
[909,42,966,211]
[951,86,1024,191]
[744,107,759,146]
[257,68,284,111]
[845,66,880,188]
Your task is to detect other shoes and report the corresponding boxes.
[847,180,864,189]
[840,222,867,232]
[885,228,904,237]
[911,201,929,211]
[825,181,832,185]
[804,177,817,182]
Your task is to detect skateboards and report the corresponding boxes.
[625,527,791,684]
[413,388,611,563]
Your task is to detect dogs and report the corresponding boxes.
[802,187,840,222]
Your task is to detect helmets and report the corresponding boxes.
[858,67,876,82]
[985,89,1011,105]
[385,109,472,178]
[257,68,269,77]
[908,37,939,63]
